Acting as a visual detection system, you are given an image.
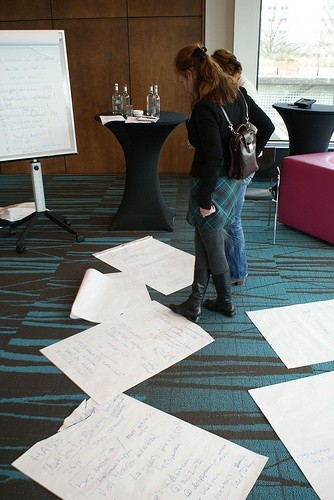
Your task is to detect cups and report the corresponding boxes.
[126,105,133,117]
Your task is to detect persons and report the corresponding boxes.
[169,43,275,323]
[211,48,258,287]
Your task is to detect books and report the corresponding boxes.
[138,115,160,123]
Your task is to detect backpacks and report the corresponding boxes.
[216,87,259,180]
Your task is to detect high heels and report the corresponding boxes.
[210,276,244,286]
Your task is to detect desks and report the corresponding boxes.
[95,111,189,233]
[273,103,334,157]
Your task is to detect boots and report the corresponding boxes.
[203,269,236,318]
[170,281,208,324]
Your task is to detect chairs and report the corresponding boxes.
[278,152,334,246]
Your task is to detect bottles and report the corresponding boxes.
[120,84,130,116]
[112,82,122,114]
[147,84,156,116]
[150,84,160,118]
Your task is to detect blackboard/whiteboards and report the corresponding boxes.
[0,29,79,162]
